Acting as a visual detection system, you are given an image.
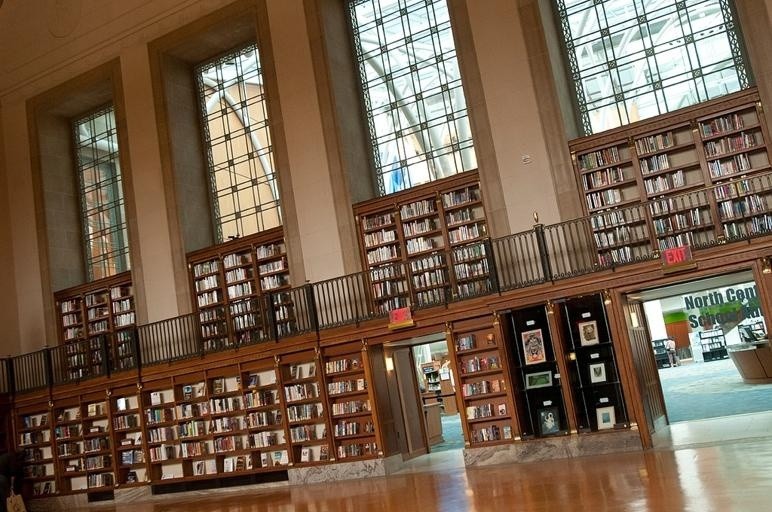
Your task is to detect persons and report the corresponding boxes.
[666,335,681,366]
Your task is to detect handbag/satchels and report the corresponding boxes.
[6,488,27,512]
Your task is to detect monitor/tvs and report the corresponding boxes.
[741,328,758,341]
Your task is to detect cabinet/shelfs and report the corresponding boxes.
[0,87,772,497]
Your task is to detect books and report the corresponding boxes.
[362,187,490,314]
[194,243,293,350]
[422,331,512,443]
[579,113,772,266]
[61,287,140,380]
